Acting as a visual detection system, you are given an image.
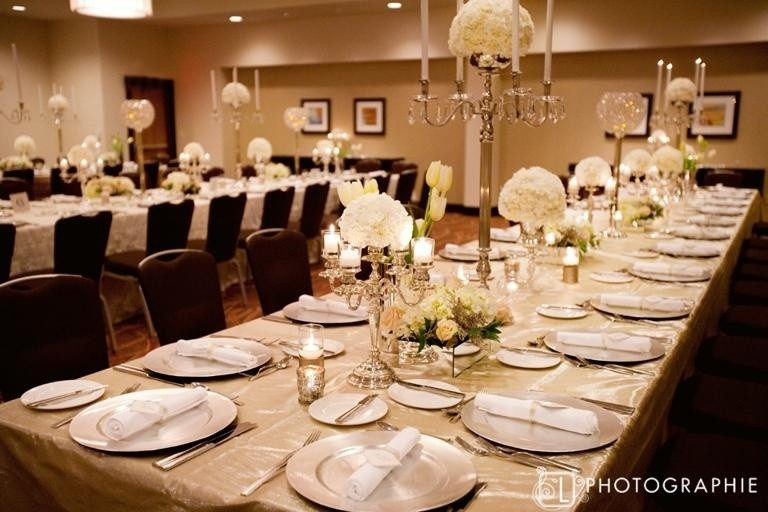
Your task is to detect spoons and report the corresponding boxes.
[191,381,244,407]
[376,421,450,441]
[446,395,476,415]
[249,355,293,380]
[563,353,633,376]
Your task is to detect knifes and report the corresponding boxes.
[160,423,258,471]
[113,365,185,388]
[478,437,582,473]
[27,384,110,409]
[280,341,334,355]
[545,305,592,310]
[335,394,379,423]
[395,380,465,398]
[500,344,562,358]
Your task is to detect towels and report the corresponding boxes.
[444,241,501,257]
[473,391,599,436]
[342,424,421,503]
[557,331,652,354]
[99,385,209,442]
[600,292,685,313]
[634,260,709,276]
[298,294,370,318]
[174,339,256,368]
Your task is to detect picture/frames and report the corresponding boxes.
[300,97,331,136]
[598,91,653,140]
[352,96,387,136]
[686,89,741,143]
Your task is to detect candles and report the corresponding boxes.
[59,86,64,106]
[254,69,262,114]
[602,177,616,198]
[699,65,707,100]
[321,231,340,258]
[694,63,700,87]
[34,83,44,117]
[296,330,325,398]
[338,243,362,276]
[510,1,521,80]
[653,64,663,115]
[542,1,554,85]
[51,84,57,113]
[69,86,79,118]
[419,0,431,85]
[408,236,435,271]
[455,0,467,87]
[210,70,219,114]
[230,67,238,85]
[567,175,580,196]
[558,246,580,281]
[666,67,670,93]
[10,43,25,107]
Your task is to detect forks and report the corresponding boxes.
[577,353,655,376]
[454,436,548,472]
[240,430,322,497]
[52,381,142,430]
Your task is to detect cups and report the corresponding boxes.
[298,355,325,370]
[504,245,536,291]
[295,364,326,405]
[296,324,325,360]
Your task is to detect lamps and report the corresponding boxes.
[119,98,156,202]
[592,91,649,238]
[569,155,612,235]
[405,1,568,287]
[655,54,707,152]
[246,136,273,179]
[315,191,435,391]
[68,0,155,21]
[178,142,211,184]
[208,64,260,181]
[494,165,583,291]
[283,106,311,177]
[31,83,79,172]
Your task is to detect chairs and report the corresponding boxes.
[240,227,313,319]
[0,220,16,285]
[193,189,250,315]
[137,247,227,349]
[105,194,197,341]
[297,181,329,241]
[393,170,419,204]
[0,272,109,405]
[241,188,293,266]
[46,208,124,357]
[361,170,389,200]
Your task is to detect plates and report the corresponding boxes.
[280,338,345,359]
[461,389,623,453]
[388,379,464,409]
[497,347,561,369]
[686,215,735,227]
[438,248,508,261]
[537,303,587,319]
[699,206,742,215]
[284,296,372,324]
[645,229,672,239]
[590,271,633,283]
[287,430,477,511]
[622,246,659,258]
[623,249,659,258]
[652,242,720,257]
[706,188,751,206]
[628,260,712,282]
[670,225,729,240]
[20,379,105,410]
[544,328,666,362]
[67,386,238,453]
[309,392,387,426]
[142,337,273,377]
[591,292,695,318]
